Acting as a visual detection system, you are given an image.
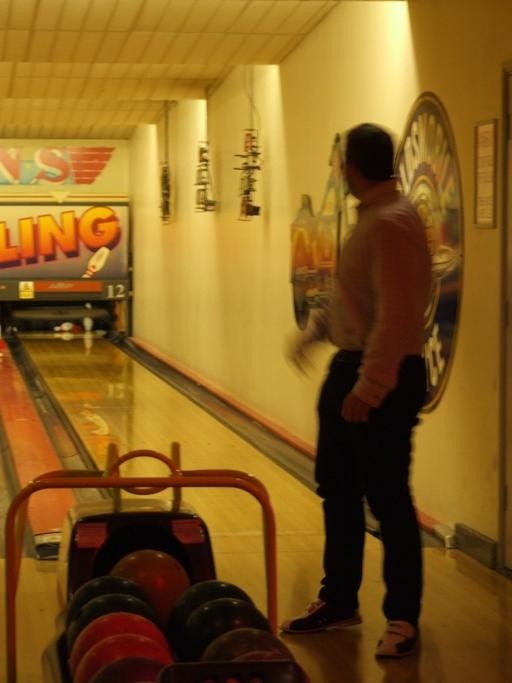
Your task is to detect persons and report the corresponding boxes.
[279,118,433,660]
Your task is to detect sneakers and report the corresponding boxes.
[375,619,420,658]
[279,598,362,633]
